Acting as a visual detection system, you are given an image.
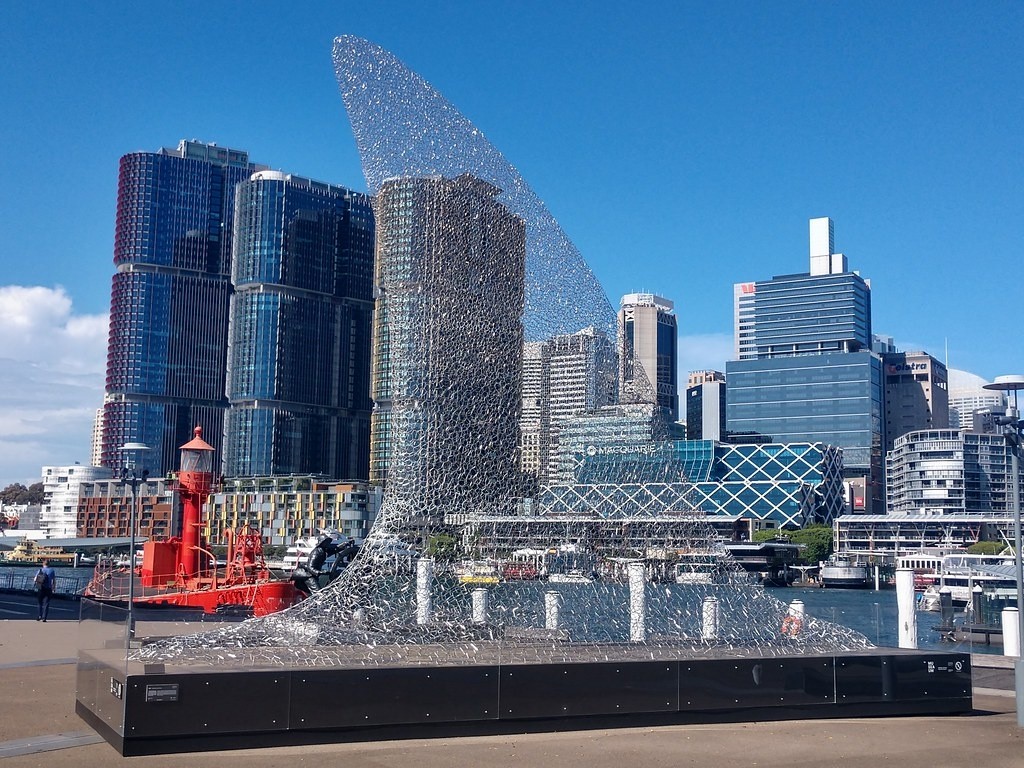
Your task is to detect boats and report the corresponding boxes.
[0,539,82,568]
[81,425,308,620]
[935,580,1009,644]
[920,555,1018,612]
[547,572,592,585]
[281,527,349,573]
[820,552,866,588]
[457,567,500,583]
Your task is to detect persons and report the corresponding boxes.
[33,560,56,623]
[290,533,355,594]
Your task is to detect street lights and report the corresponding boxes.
[982,373,1024,726]
[114,442,151,649]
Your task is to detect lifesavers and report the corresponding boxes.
[782,616,801,637]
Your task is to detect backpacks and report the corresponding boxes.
[36,569,49,591]
